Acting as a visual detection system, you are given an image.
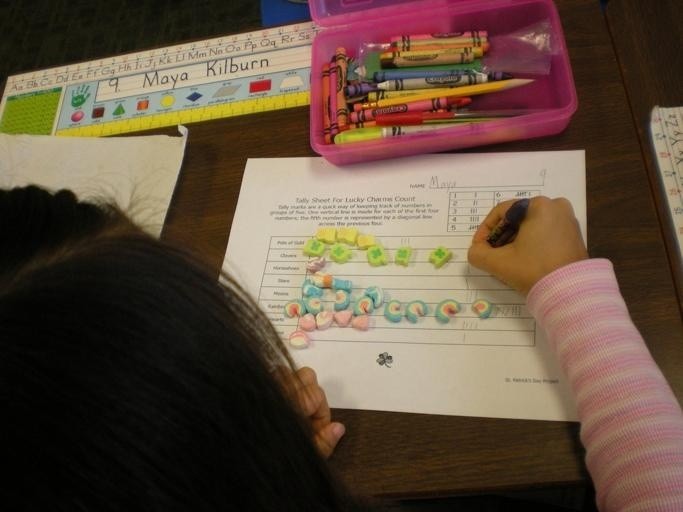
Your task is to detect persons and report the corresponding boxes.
[1,183,682,512]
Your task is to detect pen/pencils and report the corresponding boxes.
[321,30,535,144]
[487,198,531,248]
